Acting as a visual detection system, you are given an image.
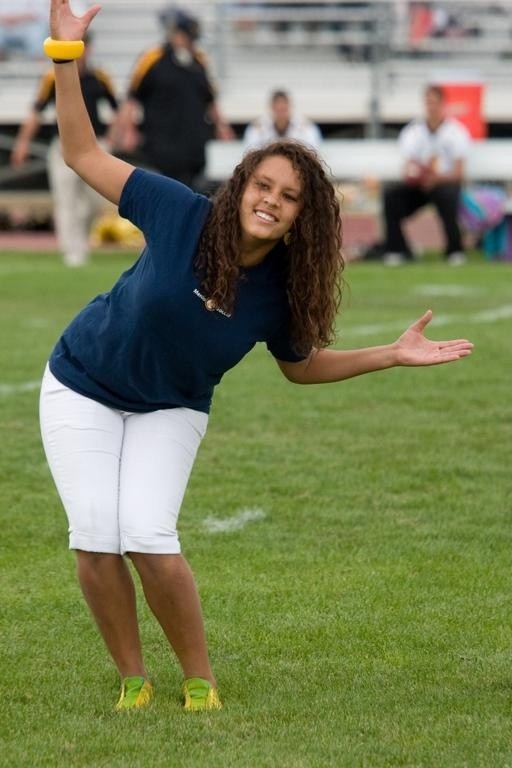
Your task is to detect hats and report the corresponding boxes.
[158,7,200,39]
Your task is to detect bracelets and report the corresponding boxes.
[42,37,86,61]
[52,58,76,64]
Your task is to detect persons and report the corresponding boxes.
[384,82,477,269]
[105,7,239,196]
[41,0,478,716]
[215,0,477,62]
[244,82,326,166]
[10,28,122,267]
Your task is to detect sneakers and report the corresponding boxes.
[445,250,469,267]
[111,676,156,712]
[63,248,91,270]
[179,677,222,714]
[381,251,403,269]
